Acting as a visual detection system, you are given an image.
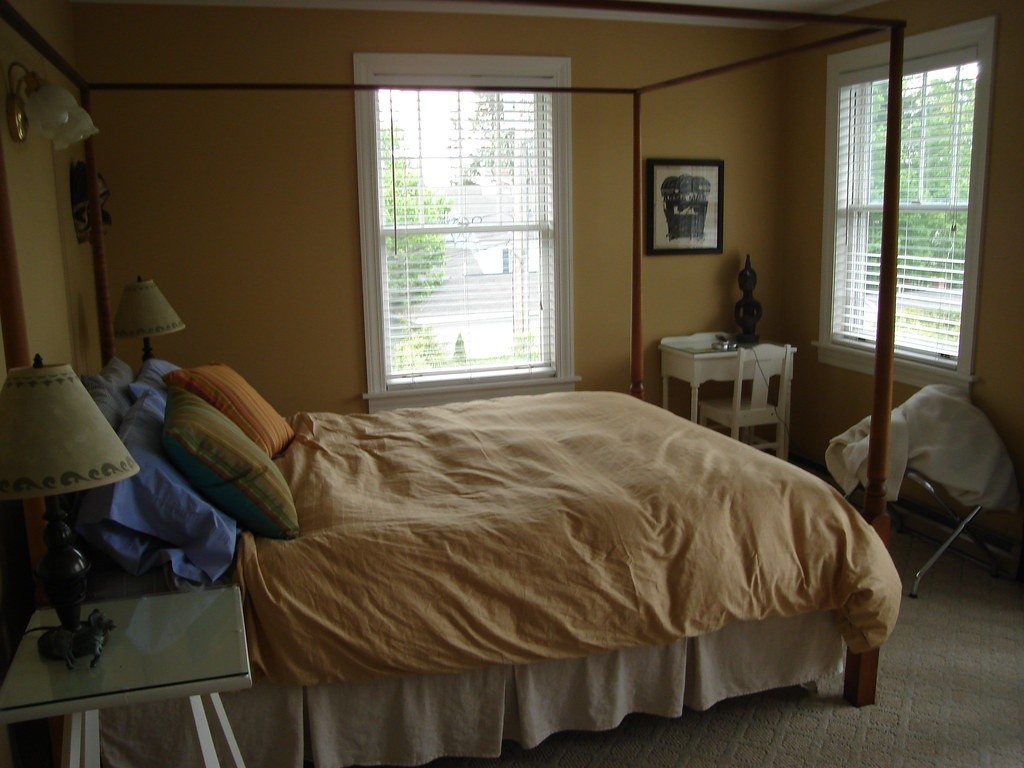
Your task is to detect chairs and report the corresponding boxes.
[825,384,1019,599]
[700,343,792,461]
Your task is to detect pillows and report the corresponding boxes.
[161,384,300,540]
[162,361,295,459]
[134,358,182,394]
[41,374,130,579]
[99,356,135,407]
[76,383,236,585]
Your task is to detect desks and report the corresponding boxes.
[659,332,797,462]
[0,586,252,768]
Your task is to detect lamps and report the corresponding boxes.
[7,62,101,152]
[112,276,185,364]
[0,353,141,668]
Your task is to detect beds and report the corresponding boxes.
[41,356,903,768]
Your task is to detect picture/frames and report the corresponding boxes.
[647,157,724,255]
